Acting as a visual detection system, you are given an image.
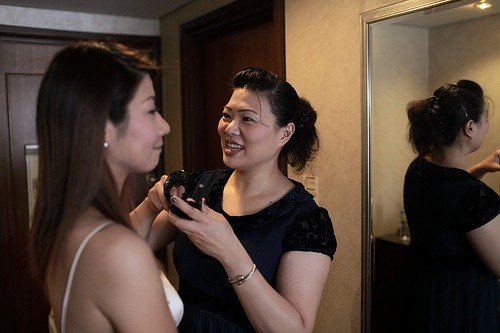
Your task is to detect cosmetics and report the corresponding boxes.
[164,170,201,220]
[397,209,410,241]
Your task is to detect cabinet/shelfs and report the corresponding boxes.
[375,230,410,333]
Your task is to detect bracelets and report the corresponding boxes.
[229,264,256,285]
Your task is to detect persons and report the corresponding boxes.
[403,79,500,333]
[129,67,337,333]
[28,40,185,333]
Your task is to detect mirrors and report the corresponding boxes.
[360,0,500,333]
